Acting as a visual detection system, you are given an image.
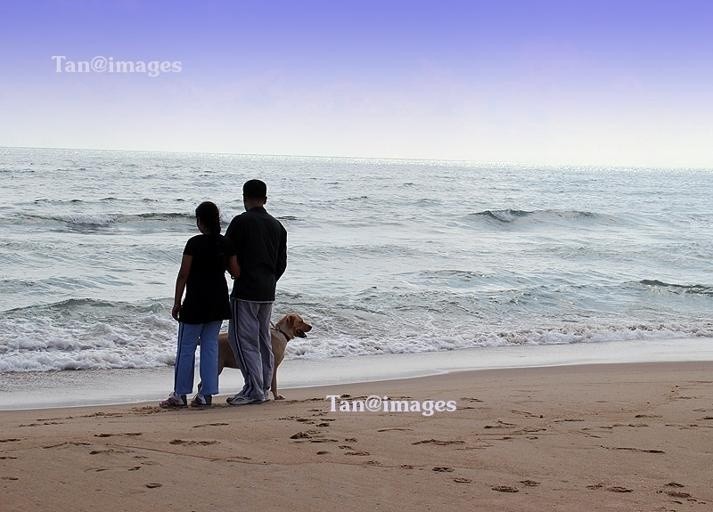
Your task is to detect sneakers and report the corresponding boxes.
[190,393,213,407]
[225,390,275,405]
[158,394,188,408]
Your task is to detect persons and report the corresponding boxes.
[225,178,288,406]
[156,199,234,410]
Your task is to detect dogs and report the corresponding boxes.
[198,313,312,400]
